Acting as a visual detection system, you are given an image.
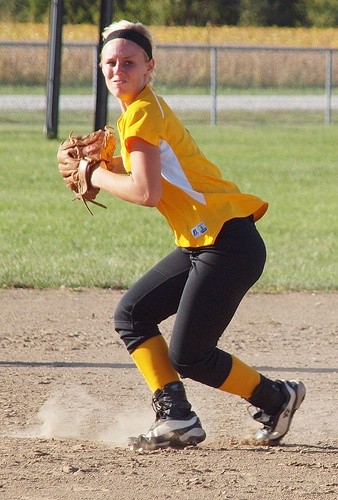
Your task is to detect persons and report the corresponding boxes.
[57,20,306,453]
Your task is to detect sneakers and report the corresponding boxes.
[253,378,306,445]
[127,411,206,448]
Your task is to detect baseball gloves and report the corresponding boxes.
[57,126,117,215]
